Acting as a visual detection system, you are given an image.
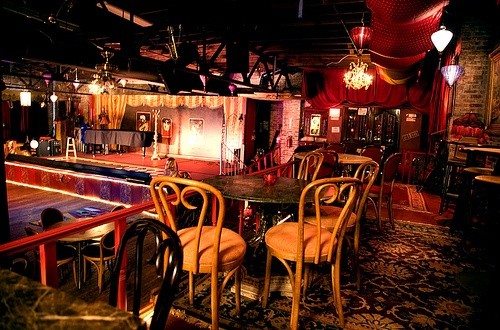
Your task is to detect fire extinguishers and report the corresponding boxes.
[287,136,292,148]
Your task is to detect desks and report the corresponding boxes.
[0,269,147,330]
[458,147,500,176]
[47,217,117,290]
[80,128,162,158]
[201,176,321,301]
[292,152,372,177]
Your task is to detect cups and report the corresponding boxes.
[264,173,277,185]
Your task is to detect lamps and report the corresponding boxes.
[342,12,373,90]
[431,18,453,54]
[441,56,463,87]
[88,52,122,94]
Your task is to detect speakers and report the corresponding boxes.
[36,140,60,156]
[226,42,249,74]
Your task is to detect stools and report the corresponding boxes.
[449,167,493,235]
[66,136,77,158]
[466,176,500,238]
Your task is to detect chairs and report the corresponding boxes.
[0,146,401,330]
[440,140,483,216]
